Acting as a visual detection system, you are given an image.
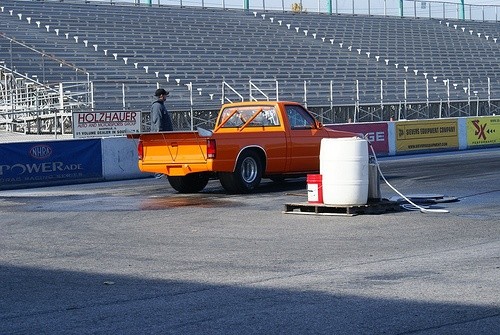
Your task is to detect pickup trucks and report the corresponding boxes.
[128,101,358,192]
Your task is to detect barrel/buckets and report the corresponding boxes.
[319,137,369,204]
[305,174,323,204]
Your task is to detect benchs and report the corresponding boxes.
[0,0,500,134]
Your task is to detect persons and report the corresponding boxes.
[150,88,173,180]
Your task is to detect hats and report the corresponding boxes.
[155,88,169,96]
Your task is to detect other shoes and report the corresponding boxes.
[155,173,164,179]
